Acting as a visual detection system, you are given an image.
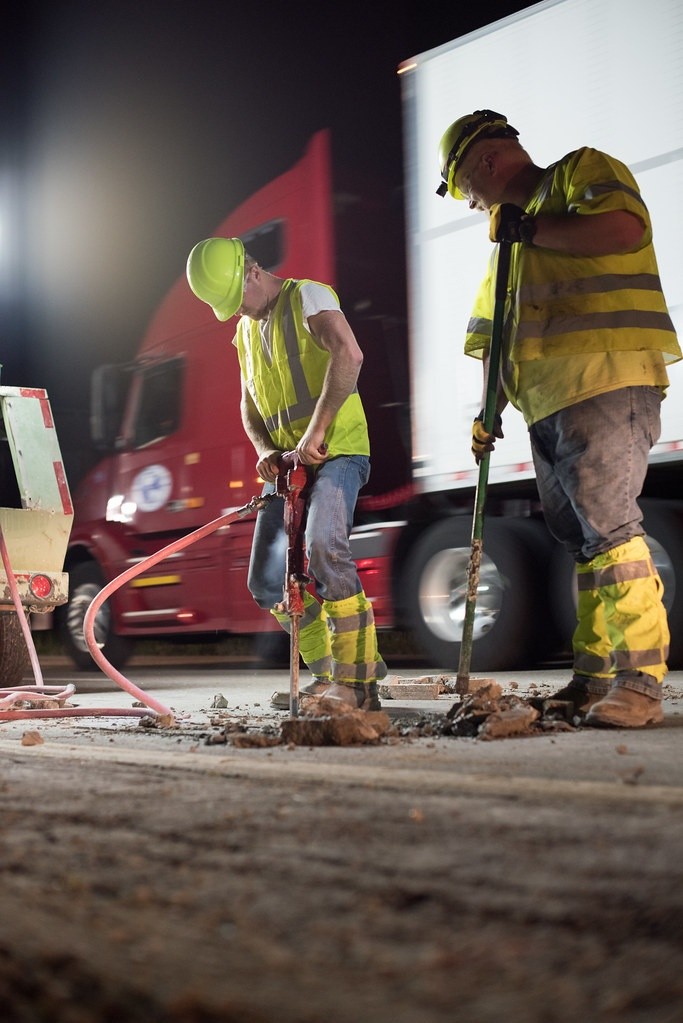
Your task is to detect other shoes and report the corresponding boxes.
[299,683,381,714]
[547,681,606,714]
[587,686,665,727]
[271,680,330,708]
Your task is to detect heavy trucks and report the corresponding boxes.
[54,1,682,674]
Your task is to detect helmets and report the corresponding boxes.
[187,237,244,321]
[436,110,508,199]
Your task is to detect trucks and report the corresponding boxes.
[0,386,76,685]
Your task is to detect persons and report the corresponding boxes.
[436,109,679,730]
[186,236,390,715]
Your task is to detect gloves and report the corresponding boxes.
[472,409,505,461]
[488,203,530,242]
